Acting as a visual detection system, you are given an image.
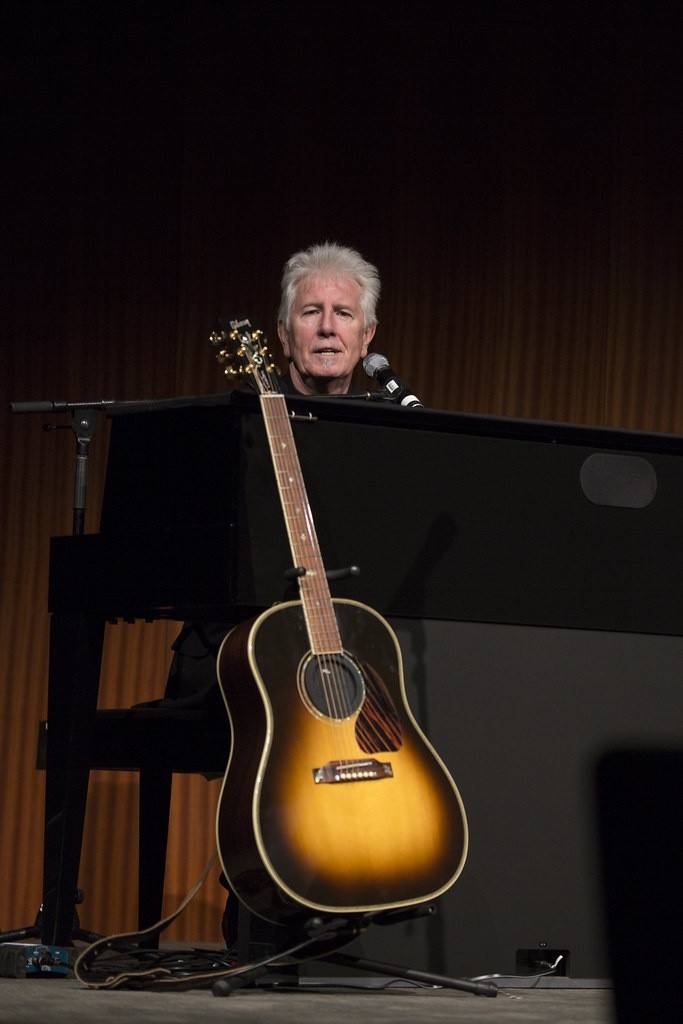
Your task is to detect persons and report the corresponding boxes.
[165,242,393,950]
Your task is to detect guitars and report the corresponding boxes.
[206,314,471,933]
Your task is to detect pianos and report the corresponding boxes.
[0,387,683,997]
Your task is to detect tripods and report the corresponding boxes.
[0,400,502,1002]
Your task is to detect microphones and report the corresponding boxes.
[364,353,424,408]
